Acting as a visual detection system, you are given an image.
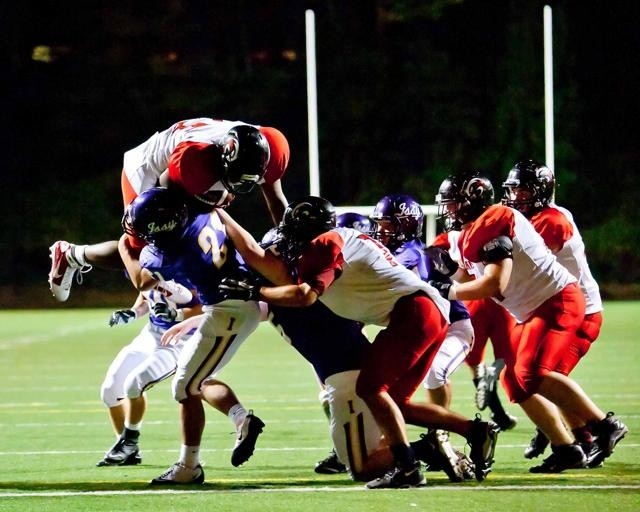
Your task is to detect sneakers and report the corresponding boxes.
[367,461,427,488]
[231,410,265,466]
[524,429,550,458]
[48,240,93,302]
[315,454,347,473]
[97,439,141,466]
[150,463,204,485]
[530,411,629,473]
[420,428,473,482]
[476,362,496,410]
[464,413,515,482]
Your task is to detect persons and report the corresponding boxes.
[96,186,268,486]
[48,118,291,302]
[427,162,627,474]
[214,191,500,487]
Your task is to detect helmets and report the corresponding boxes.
[501,160,554,214]
[278,197,335,255]
[337,213,373,236]
[121,187,188,244]
[368,194,423,248]
[220,125,270,196]
[435,172,494,233]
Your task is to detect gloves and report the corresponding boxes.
[218,277,261,301]
[425,245,458,278]
[152,302,177,319]
[109,310,136,326]
